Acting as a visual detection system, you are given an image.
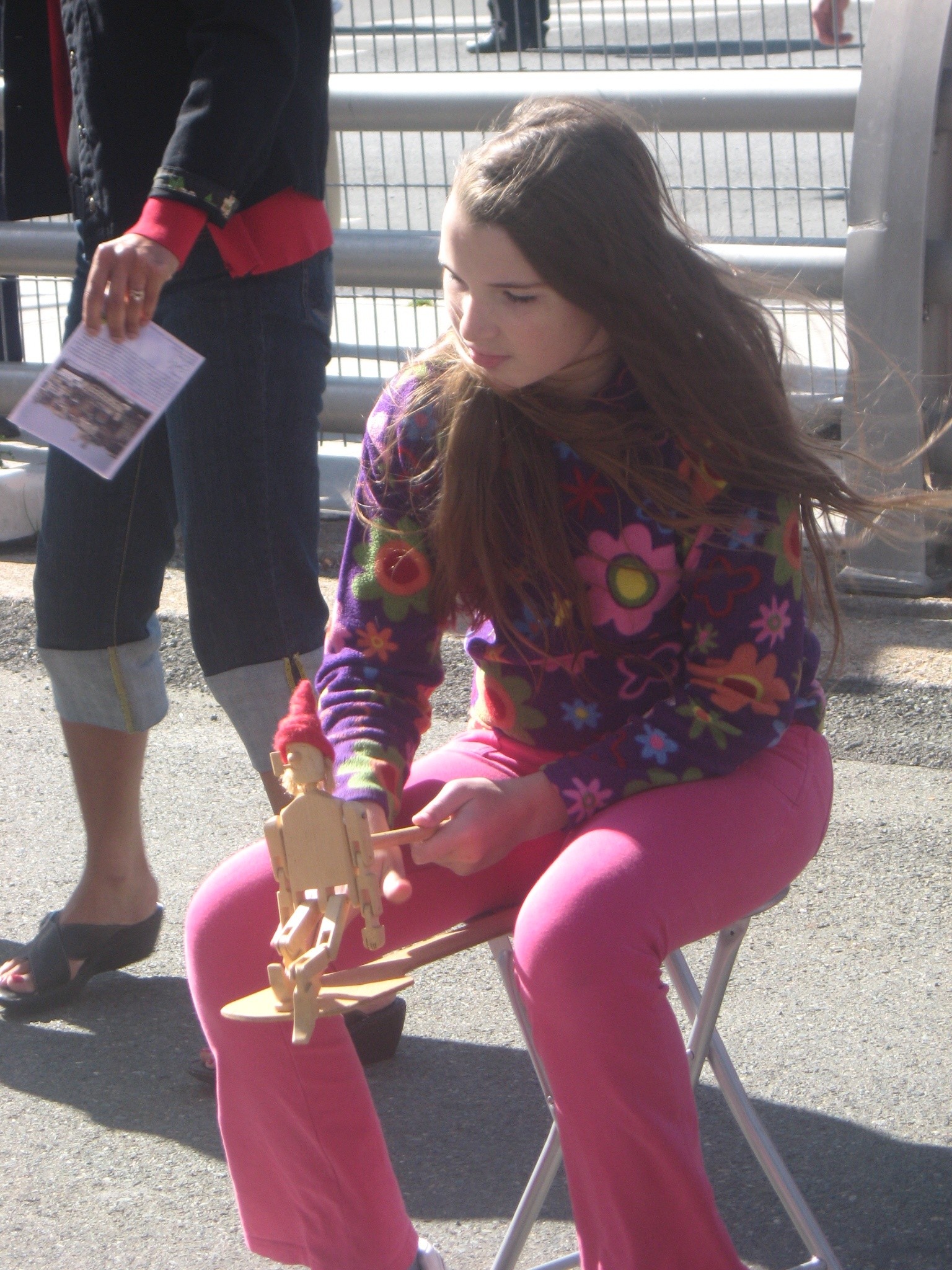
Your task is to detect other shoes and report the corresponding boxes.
[465,27,546,53]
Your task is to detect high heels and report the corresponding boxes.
[1,897,163,1014]
[185,998,407,1086]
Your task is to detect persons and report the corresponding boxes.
[186,91,952,1270]
[810,1,854,46]
[467,1,551,54]
[2,0,404,1083]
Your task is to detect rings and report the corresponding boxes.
[127,289,145,302]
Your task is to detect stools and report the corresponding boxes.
[487,880,840,1270]
